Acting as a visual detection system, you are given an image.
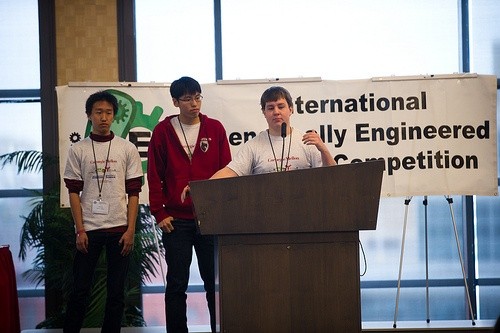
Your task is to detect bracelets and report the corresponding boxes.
[77,229,85,234]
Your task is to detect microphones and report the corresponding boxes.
[281,122,287,172]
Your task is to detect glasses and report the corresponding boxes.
[173,94,203,102]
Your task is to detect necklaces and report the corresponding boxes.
[268,127,292,172]
[178,114,193,157]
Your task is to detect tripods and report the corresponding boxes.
[393,194,476,328]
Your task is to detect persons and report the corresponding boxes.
[63,92,143,333]
[147,77,232,333]
[181,86,338,203]
